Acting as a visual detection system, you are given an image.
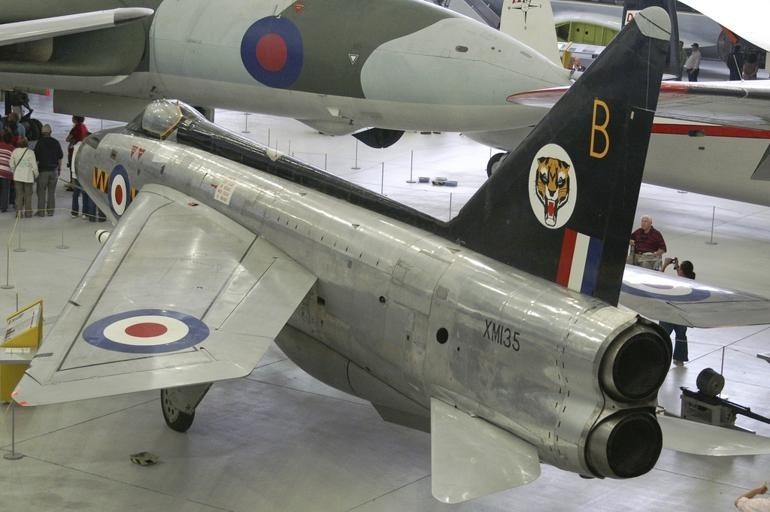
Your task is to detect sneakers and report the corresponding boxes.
[16,211,54,218]
[71,212,106,222]
[674,361,684,367]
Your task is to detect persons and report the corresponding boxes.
[629,214,667,273]
[87,196,107,223]
[69,131,93,219]
[569,55,587,72]
[658,255,696,368]
[679,39,688,80]
[741,52,758,79]
[685,42,702,82]
[727,44,745,80]
[0,104,64,217]
[65,113,89,191]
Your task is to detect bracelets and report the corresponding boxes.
[673,265,679,270]
[654,251,657,257]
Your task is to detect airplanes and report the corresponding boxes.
[1,3,769,205]
[1,6,770,504]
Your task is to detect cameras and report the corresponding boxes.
[670,259,676,264]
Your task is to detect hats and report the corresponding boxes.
[73,112,88,117]
[41,124,51,132]
